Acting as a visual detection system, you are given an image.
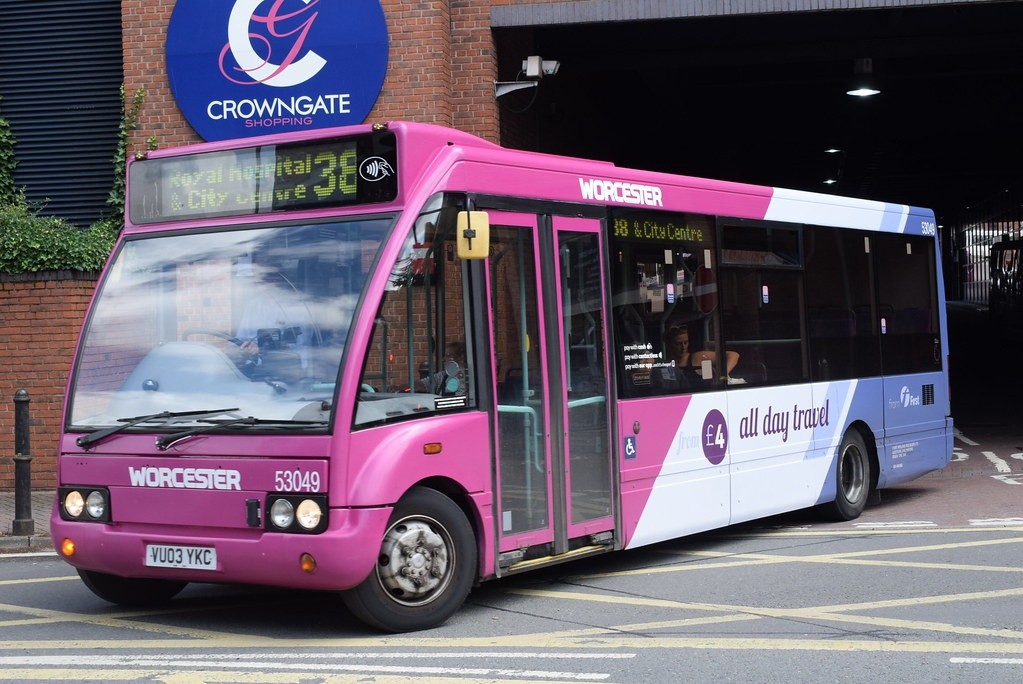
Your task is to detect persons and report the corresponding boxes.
[386,340,467,396]
[223,262,314,389]
[624,322,740,397]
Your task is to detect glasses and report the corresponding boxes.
[667,325,688,340]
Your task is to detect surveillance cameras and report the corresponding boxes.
[522,60,560,75]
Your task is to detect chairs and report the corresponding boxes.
[741,356,832,386]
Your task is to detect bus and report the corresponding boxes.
[48,120,956,636]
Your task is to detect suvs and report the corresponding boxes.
[966,231,1009,258]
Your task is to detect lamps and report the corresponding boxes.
[845,57,883,97]
